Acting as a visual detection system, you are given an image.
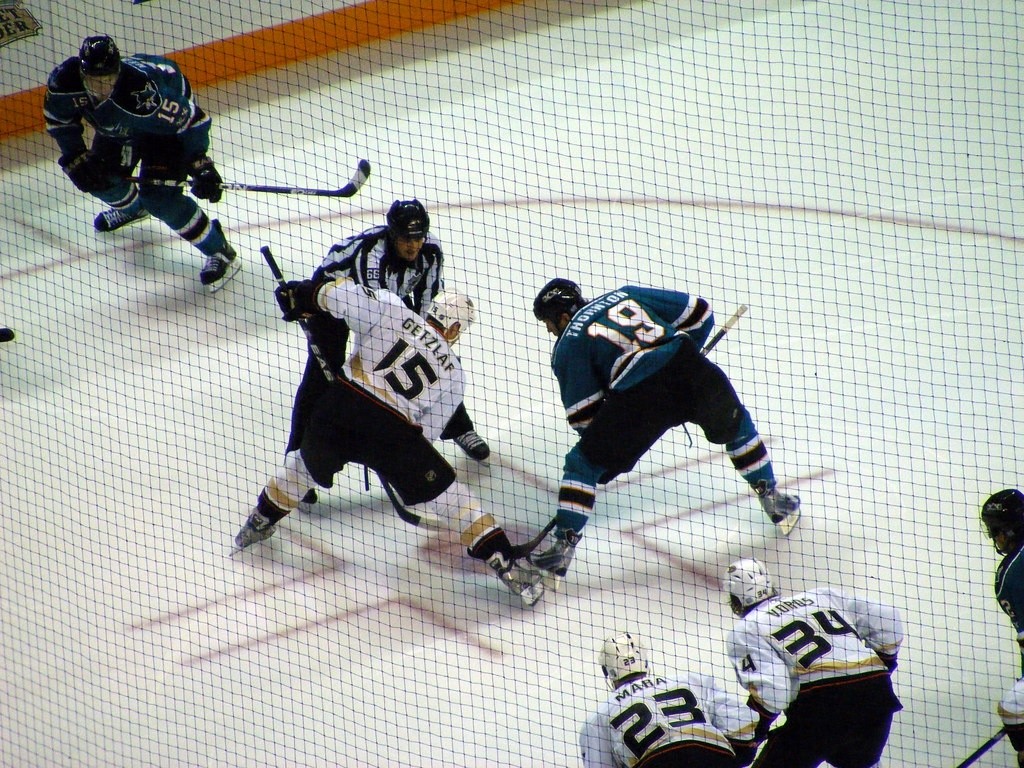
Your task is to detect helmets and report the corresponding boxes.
[388,197,430,241]
[80,33,122,77]
[431,287,476,333]
[979,489,1024,557]
[722,557,776,618]
[598,633,648,682]
[533,278,583,325]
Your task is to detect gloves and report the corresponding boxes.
[741,695,781,748]
[726,736,758,768]
[186,157,222,203]
[877,652,898,676]
[275,278,333,321]
[59,145,113,194]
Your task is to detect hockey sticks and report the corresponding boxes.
[764,724,786,739]
[464,302,751,562]
[958,724,1007,768]
[123,157,372,198]
[258,244,455,534]
[363,465,372,493]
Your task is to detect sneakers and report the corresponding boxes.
[200,219,241,292]
[298,489,319,513]
[228,506,276,557]
[485,551,546,608]
[453,429,492,469]
[514,527,583,592]
[94,202,149,232]
[750,479,802,536]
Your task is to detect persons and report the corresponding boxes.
[977,489,1024,765]
[579,634,756,768]
[527,279,801,576]
[237,280,541,601]
[42,35,236,287]
[723,557,903,768]
[292,199,490,506]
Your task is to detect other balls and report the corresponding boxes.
[0,328,15,342]
